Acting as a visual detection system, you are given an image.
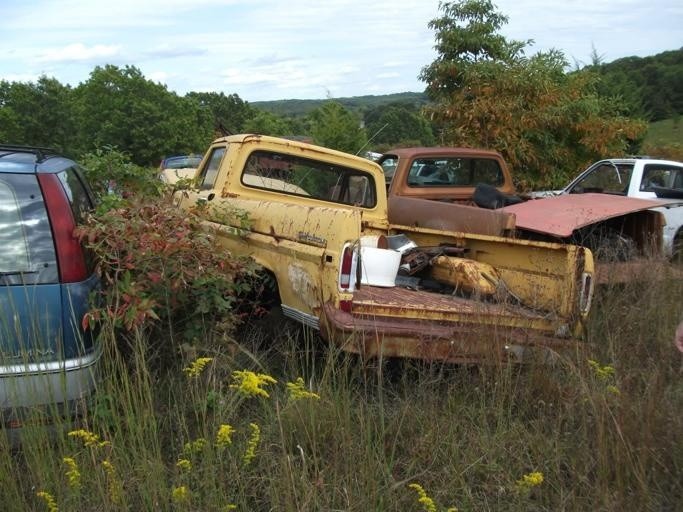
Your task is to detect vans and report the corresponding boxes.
[1,147,109,414]
